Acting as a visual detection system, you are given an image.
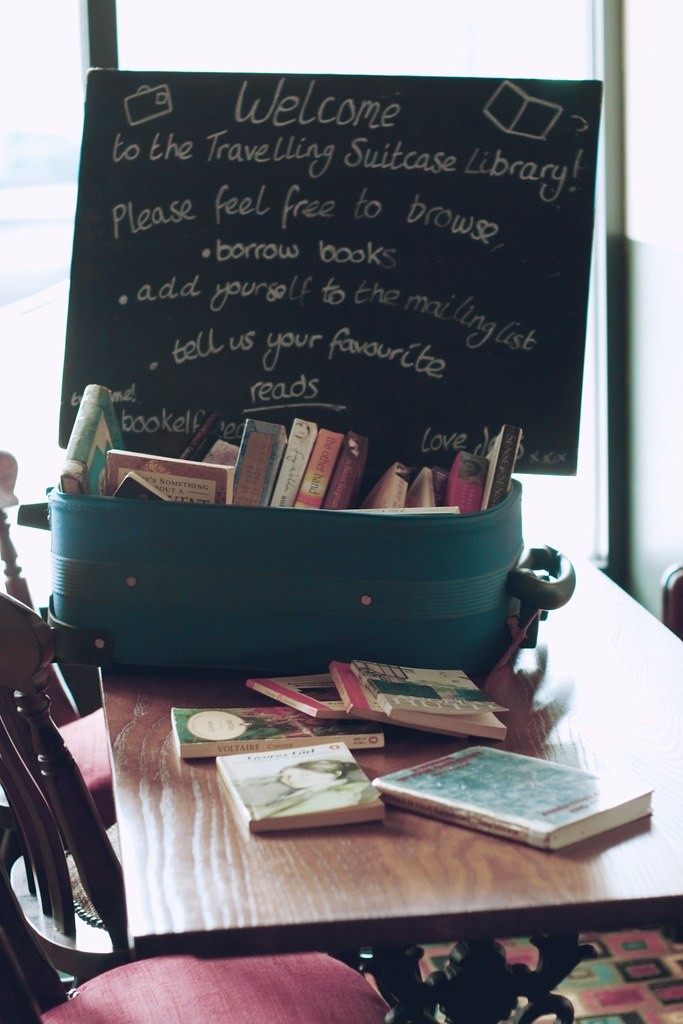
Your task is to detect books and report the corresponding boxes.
[59,384,524,518]
[168,661,653,850]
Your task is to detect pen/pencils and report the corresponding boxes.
[178,410,220,461]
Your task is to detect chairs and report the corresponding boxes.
[0,451,396,1024]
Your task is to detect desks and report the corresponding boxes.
[99,552,683,1024]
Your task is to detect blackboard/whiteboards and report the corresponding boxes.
[56,68,603,481]
